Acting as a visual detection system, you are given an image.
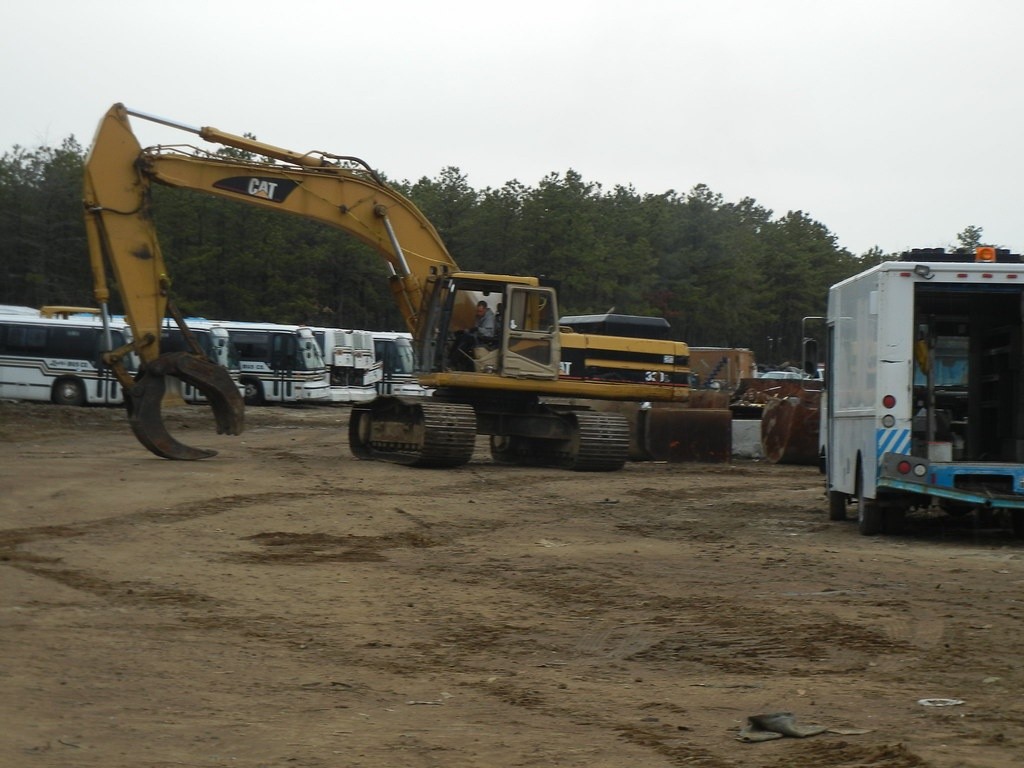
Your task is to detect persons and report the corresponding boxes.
[453,300,495,370]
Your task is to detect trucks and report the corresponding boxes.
[800,243,1024,536]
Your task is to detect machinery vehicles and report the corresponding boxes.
[730,371,824,465]
[79,96,757,474]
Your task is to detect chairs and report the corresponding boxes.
[477,301,504,348]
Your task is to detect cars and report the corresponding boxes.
[757,362,825,380]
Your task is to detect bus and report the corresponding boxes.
[2,303,438,405]
